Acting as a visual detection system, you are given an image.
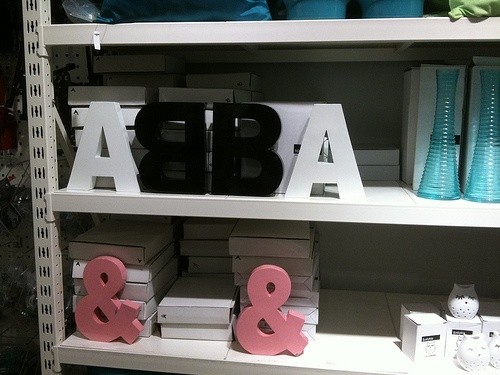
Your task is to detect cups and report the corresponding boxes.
[488,331,500,369]
[456,332,491,371]
[360,0,423,18]
[448,283,480,319]
[284,0,349,19]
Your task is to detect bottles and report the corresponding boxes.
[417,69,462,201]
[463,70,500,203]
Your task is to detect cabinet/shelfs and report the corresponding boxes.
[20,0,500,375]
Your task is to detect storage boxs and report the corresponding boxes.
[398,300,500,365]
[401,56,500,191]
[68,213,320,342]
[64,53,399,196]
